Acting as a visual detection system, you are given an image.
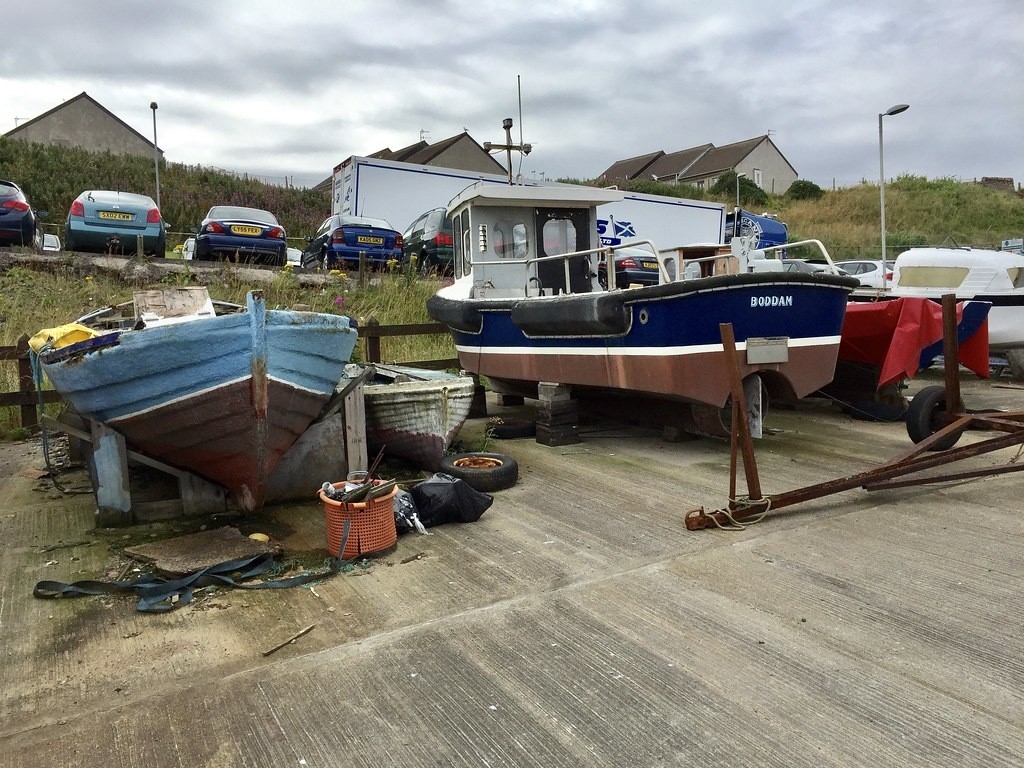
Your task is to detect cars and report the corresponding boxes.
[64,190,172,259]
[179,205,303,269]
[300,215,404,273]
[0,179,50,247]
[597,242,663,291]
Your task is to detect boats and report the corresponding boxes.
[354,362,477,471]
[422,180,859,440]
[29,284,361,525]
[843,245,1024,351]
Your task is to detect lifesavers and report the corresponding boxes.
[439,451,519,493]
[905,385,966,452]
[486,417,537,440]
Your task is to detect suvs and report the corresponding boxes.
[404,207,454,276]
[784,257,898,291]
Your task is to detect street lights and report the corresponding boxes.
[736,172,747,207]
[150,101,162,214]
[879,102,911,290]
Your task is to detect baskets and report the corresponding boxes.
[319,477,399,559]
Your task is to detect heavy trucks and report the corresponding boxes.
[330,156,789,272]
